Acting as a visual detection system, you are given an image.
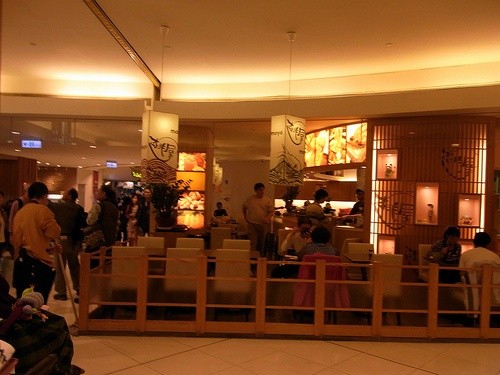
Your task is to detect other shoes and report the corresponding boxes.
[66,365,85,375]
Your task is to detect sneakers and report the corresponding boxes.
[74,298,79,302]
[54,294,66,299]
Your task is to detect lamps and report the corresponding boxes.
[267,28,309,187]
[141,24,182,183]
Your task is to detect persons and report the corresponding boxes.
[280,217,340,262]
[0,182,150,375]
[214,201,227,218]
[323,203,336,214]
[348,189,365,216]
[427,226,462,284]
[242,183,275,250]
[306,188,329,222]
[459,232,500,316]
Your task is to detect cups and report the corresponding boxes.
[0,339,16,371]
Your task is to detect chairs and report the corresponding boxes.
[108,237,500,319]
